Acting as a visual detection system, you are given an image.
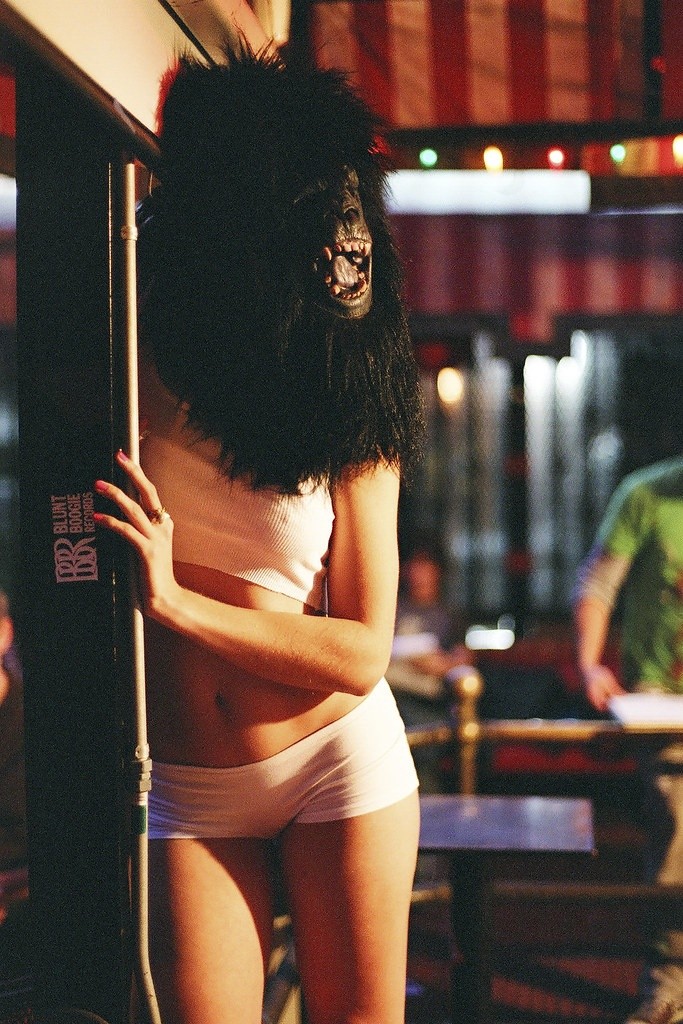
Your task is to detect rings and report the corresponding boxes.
[146,507,169,523]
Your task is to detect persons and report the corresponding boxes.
[90,46,428,1024]
[570,459,682,1024]
[386,548,479,731]
